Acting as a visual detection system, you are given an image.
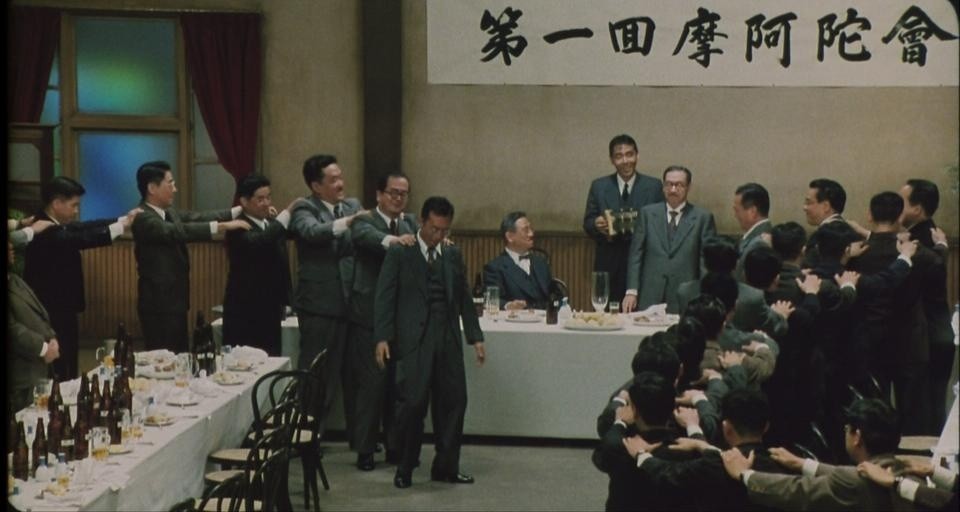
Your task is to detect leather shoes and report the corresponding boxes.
[356,451,375,471]
[384,451,421,467]
[349,439,383,452]
[431,468,474,485]
[393,468,414,490]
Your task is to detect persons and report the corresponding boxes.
[582,134,667,312]
[621,166,717,313]
[594,177,960,512]
[7,157,485,489]
[481,210,565,314]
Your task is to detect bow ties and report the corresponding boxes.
[518,253,532,262]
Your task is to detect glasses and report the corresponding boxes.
[384,189,409,201]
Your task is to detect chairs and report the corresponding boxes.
[787,372,893,462]
[169,348,330,512]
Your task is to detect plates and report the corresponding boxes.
[215,357,259,387]
[566,316,625,329]
[628,310,680,326]
[137,351,216,434]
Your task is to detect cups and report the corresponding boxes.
[486,286,500,314]
[610,300,621,318]
[31,376,52,408]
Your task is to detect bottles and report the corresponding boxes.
[190,311,217,374]
[506,307,545,323]
[547,278,559,324]
[114,323,135,384]
[76,371,132,447]
[471,270,484,319]
[10,402,90,482]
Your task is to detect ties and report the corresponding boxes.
[621,182,630,201]
[667,211,679,244]
[389,219,397,235]
[332,205,341,219]
[427,247,436,263]
[163,210,174,225]
[263,221,268,229]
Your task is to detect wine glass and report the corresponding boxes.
[590,270,609,319]
[556,296,573,325]
